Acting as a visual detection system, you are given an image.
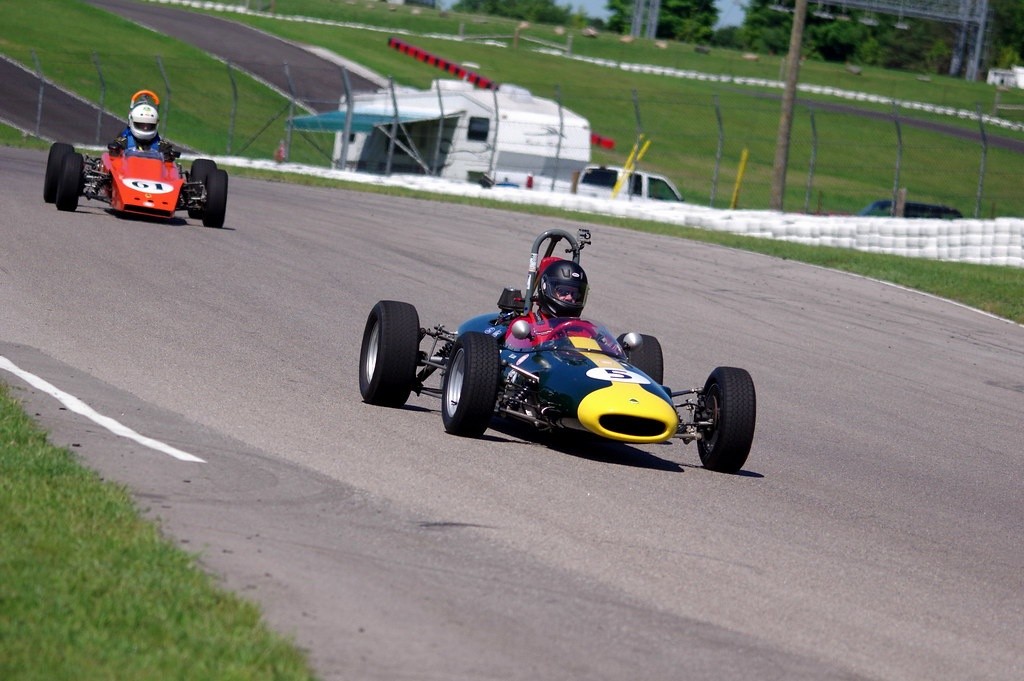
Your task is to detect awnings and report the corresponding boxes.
[285,107,418,133]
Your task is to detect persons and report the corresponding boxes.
[503,258,627,366]
[107,101,177,170]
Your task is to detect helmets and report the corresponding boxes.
[538,260,589,319]
[128,104,159,139]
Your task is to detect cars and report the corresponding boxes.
[861,199,965,222]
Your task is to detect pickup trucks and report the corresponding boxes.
[527,164,686,207]
